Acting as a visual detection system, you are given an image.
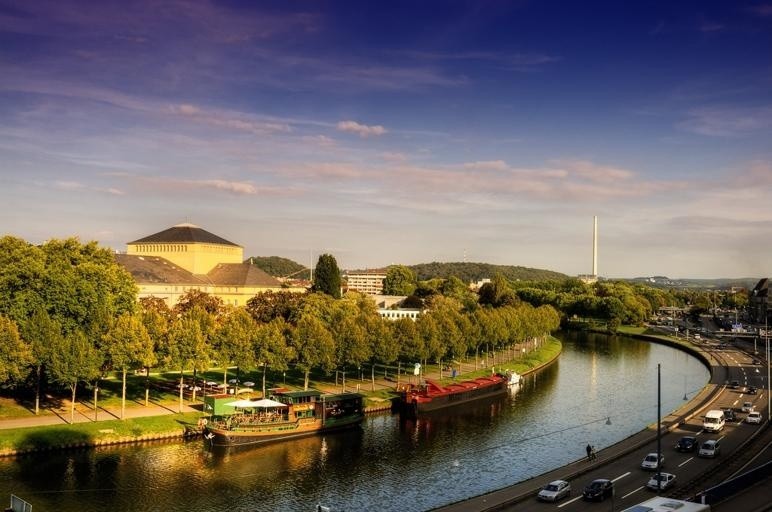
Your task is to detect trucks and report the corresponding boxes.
[618,496,711,512]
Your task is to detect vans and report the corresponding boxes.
[703,410,726,433]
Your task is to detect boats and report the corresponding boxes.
[507,371,524,386]
[394,373,510,416]
[199,390,368,448]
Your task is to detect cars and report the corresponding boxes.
[746,412,763,425]
[741,401,754,413]
[720,407,737,422]
[537,480,572,503]
[675,436,699,454]
[582,479,614,501]
[692,327,727,351]
[698,440,721,458]
[647,473,677,491]
[751,358,761,365]
[731,381,740,389]
[748,386,758,396]
[641,451,666,471]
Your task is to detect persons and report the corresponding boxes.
[591,445,598,460]
[586,443,592,459]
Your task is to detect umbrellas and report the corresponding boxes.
[176,379,256,392]
